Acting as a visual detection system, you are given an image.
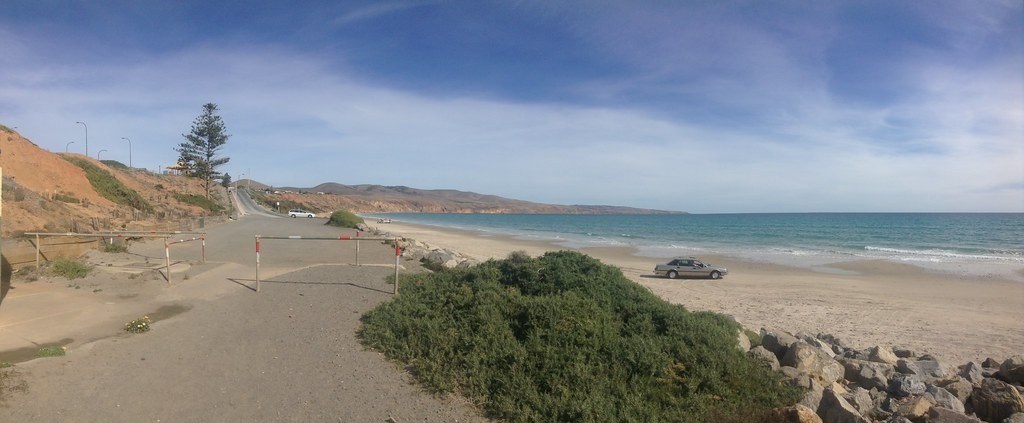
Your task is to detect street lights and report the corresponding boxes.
[77,121,87,157]
[122,137,131,166]
[66,141,75,152]
[98,149,107,161]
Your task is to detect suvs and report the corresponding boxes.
[288,208,316,218]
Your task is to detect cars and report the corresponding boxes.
[653,255,729,280]
